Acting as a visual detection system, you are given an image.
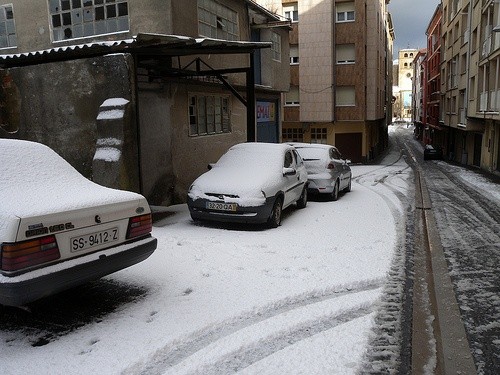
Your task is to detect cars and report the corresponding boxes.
[1,138,158,313]
[187,142,352,227]
